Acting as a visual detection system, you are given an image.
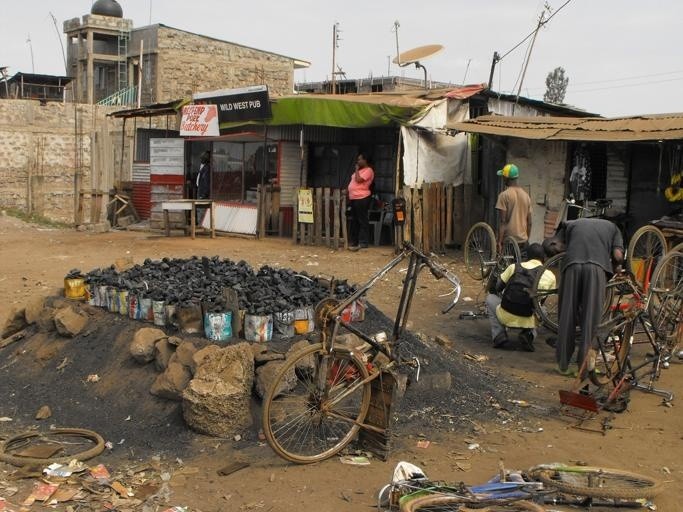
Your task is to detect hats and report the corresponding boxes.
[497,164,519,177]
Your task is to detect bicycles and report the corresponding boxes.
[459,221,522,320]
[531,196,682,414]
[261,240,463,466]
[377,460,664,511]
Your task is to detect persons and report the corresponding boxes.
[542,218,625,378]
[194,150,210,225]
[495,164,533,261]
[347,152,374,251]
[485,241,557,352]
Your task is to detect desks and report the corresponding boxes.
[160,198,214,240]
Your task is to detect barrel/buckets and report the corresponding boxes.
[631,256,644,282]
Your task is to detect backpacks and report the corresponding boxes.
[501,263,546,317]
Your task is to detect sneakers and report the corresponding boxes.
[493,330,508,348]
[518,328,534,352]
